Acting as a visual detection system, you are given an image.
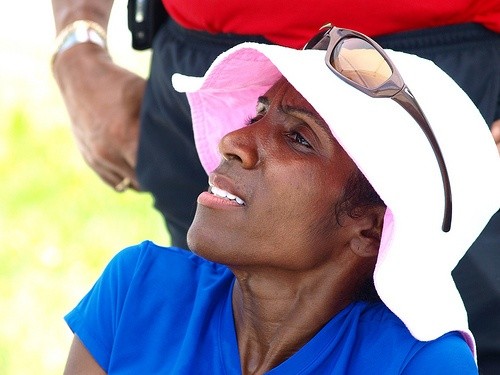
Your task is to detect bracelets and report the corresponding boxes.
[51,19,107,61]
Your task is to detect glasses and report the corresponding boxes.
[300,22,453,232]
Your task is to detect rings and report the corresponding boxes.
[117,177,130,191]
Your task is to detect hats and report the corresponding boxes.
[171,42,499,367]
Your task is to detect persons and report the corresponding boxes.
[49,0,500,375]
[62,42,500,375]
